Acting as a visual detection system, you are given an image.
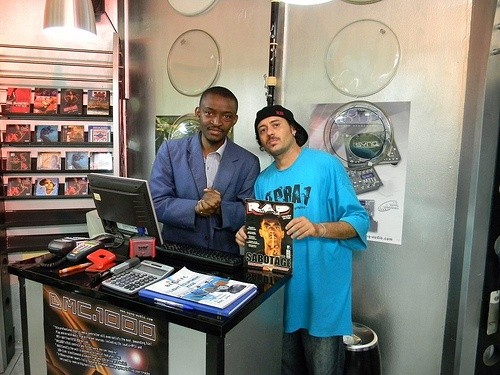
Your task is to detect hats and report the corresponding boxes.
[254,104,309,149]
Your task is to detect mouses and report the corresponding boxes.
[92,234,115,244]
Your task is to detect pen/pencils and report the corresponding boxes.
[59,262,91,276]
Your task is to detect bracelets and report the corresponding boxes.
[319,223,327,238]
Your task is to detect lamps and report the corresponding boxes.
[35,0,117,35]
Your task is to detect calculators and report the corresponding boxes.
[101,258,174,295]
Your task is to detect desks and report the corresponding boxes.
[8,236,292,375]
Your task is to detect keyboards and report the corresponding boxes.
[155,240,244,267]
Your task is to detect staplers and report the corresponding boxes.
[109,257,141,276]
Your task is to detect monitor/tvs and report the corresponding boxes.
[87,173,163,248]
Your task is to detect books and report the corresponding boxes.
[144,266,255,309]
[138,270,259,317]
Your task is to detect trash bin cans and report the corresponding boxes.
[336,321,382,375]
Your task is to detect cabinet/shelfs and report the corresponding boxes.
[0,34,119,247]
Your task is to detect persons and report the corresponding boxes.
[39,178,57,196]
[235,105,371,374]
[148,86,260,255]
[258,214,285,258]
[62,89,79,115]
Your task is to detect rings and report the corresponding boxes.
[200,210,202,214]
